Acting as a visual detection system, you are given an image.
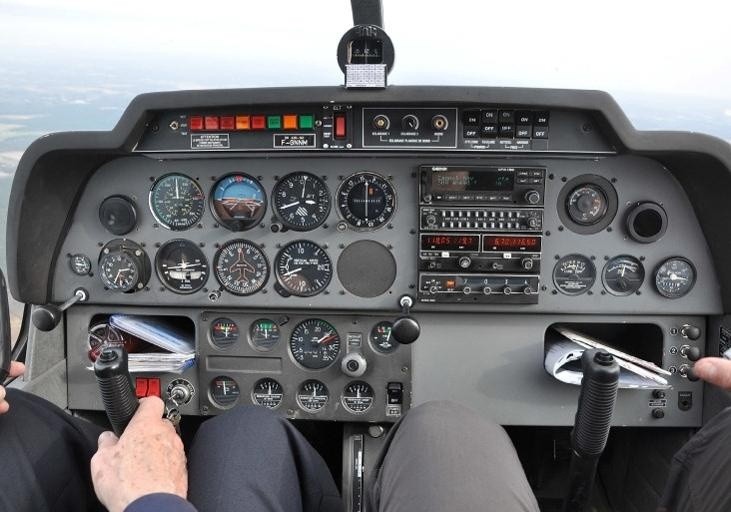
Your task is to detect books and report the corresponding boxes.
[555,327,672,384]
[110,314,194,352]
[545,341,671,390]
[128,356,195,374]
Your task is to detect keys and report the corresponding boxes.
[164,392,184,437]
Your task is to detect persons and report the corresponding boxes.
[366,356,731,512]
[0,361,341,512]
